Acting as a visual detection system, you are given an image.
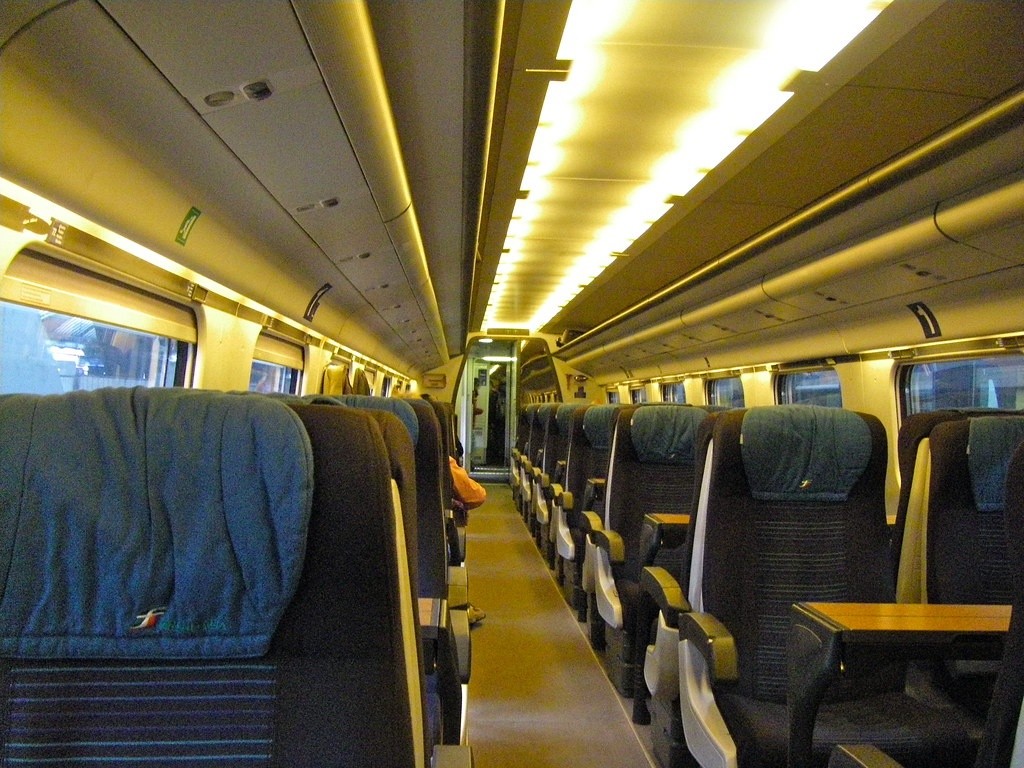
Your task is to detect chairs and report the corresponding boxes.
[0,386,472,768]
[506,403,1024,768]
